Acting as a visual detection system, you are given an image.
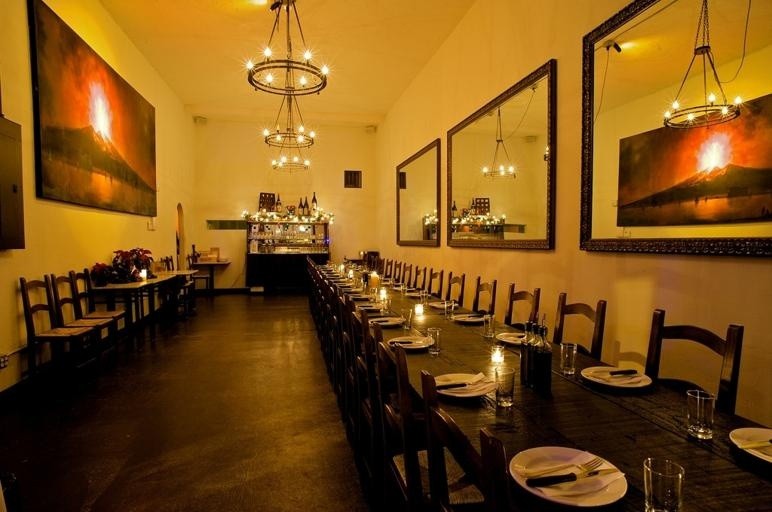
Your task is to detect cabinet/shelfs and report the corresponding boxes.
[452,219,504,240]
[422,217,437,240]
[239,207,331,297]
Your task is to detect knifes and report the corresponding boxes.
[525,467,619,488]
[591,369,637,377]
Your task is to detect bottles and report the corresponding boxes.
[452,197,477,217]
[276,191,318,215]
[526,324,541,388]
[519,322,535,384]
[533,326,553,390]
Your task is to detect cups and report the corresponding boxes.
[370,288,378,303]
[400,284,408,297]
[643,458,685,512]
[401,308,412,329]
[495,366,516,406]
[420,290,428,309]
[316,261,419,291]
[444,301,454,320]
[247,231,328,254]
[383,298,392,315]
[427,327,441,355]
[483,315,496,338]
[686,390,716,439]
[452,232,503,240]
[560,342,577,376]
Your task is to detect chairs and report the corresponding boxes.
[186,253,212,303]
[19,250,200,428]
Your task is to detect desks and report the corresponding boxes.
[191,259,232,301]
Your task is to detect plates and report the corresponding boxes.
[357,304,381,310]
[428,302,459,309]
[434,373,497,397]
[495,332,527,346]
[452,314,496,323]
[387,336,435,350]
[729,428,771,462]
[407,292,431,299]
[509,447,628,507]
[349,294,369,300]
[580,366,653,388]
[368,317,406,326]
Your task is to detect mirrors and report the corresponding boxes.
[395,137,441,247]
[578,1,772,258]
[447,58,557,250]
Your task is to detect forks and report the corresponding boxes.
[435,374,490,391]
[519,456,604,478]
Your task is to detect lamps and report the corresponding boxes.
[480,106,517,180]
[663,1,744,130]
[243,0,330,174]
